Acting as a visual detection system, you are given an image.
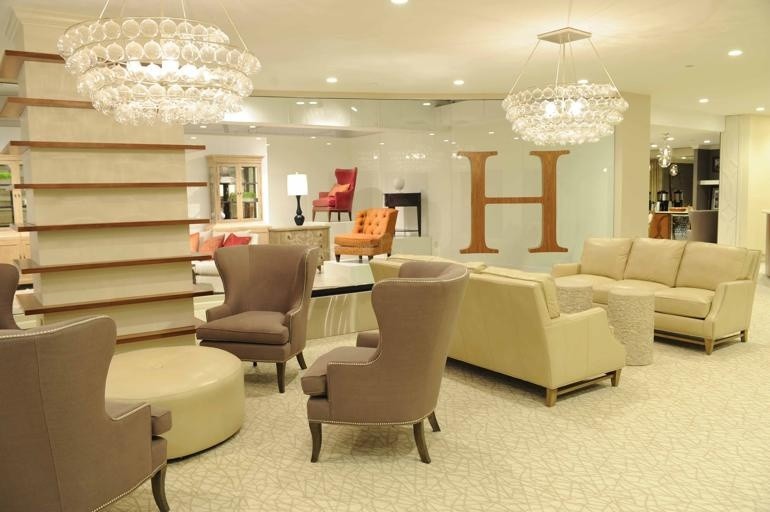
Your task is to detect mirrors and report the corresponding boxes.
[220,167,257,219]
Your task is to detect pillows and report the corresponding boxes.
[190,234,252,253]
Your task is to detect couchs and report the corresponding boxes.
[189,230,261,285]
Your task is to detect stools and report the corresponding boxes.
[100,345,246,458]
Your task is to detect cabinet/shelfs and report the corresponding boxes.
[205,155,266,227]
[0,49,214,357]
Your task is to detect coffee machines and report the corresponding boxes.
[657,190,669,211]
[672,190,682,206]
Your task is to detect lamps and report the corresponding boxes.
[287,171,308,225]
[56,2,261,126]
[502,1,631,148]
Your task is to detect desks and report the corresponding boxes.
[269,226,331,274]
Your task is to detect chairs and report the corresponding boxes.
[333,208,399,262]
[312,167,357,221]
[0,265,172,504]
